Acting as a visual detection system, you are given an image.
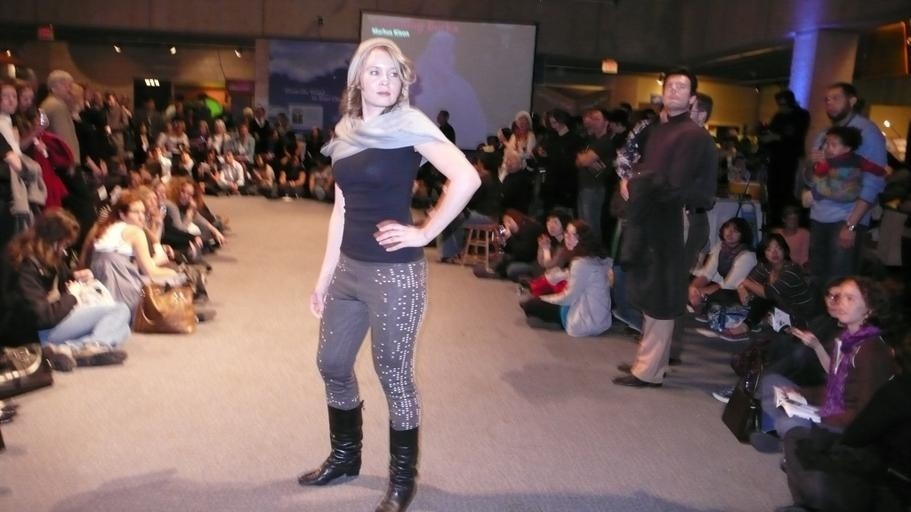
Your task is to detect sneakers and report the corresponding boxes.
[694,314,709,323]
[42,343,78,371]
[720,329,750,342]
[73,341,127,366]
[712,385,736,404]
[696,327,719,338]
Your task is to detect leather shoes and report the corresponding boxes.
[750,430,783,453]
[619,365,666,378]
[612,375,663,387]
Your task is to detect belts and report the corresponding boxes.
[686,208,705,216]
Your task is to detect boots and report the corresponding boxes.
[299,400,364,485]
[375,420,418,512]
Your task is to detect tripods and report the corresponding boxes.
[735,159,768,242]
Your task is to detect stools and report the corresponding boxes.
[461,217,502,273]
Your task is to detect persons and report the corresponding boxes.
[439,65,909,512]
[216,101,458,213]
[296,33,481,512]
[1,64,234,375]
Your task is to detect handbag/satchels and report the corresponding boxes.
[723,364,764,443]
[1,342,52,400]
[132,284,198,334]
[707,303,763,332]
